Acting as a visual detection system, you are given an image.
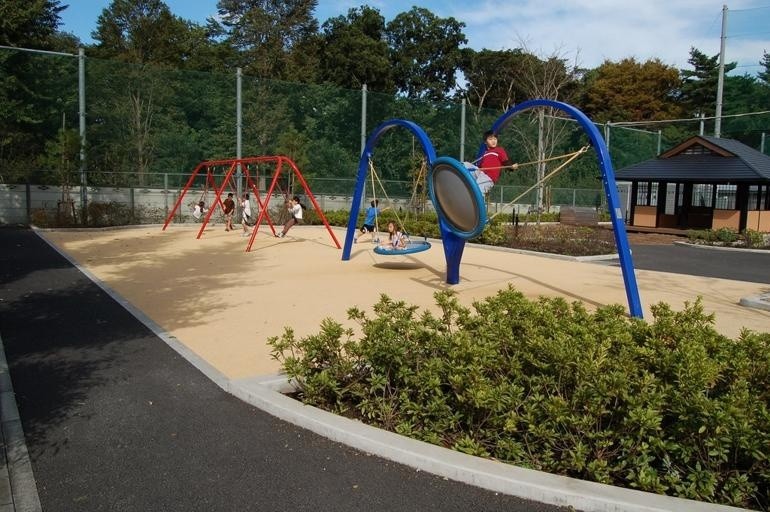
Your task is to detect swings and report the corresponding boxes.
[369,159,432,255]
[268,160,304,225]
[191,163,215,223]
[427,142,589,240]
[223,163,258,225]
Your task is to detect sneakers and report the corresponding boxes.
[275,232,284,237]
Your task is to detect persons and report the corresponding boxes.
[222,193,235,231]
[462,130,519,198]
[273,196,307,238]
[354,200,391,244]
[237,193,251,237]
[379,221,407,251]
[191,201,209,222]
